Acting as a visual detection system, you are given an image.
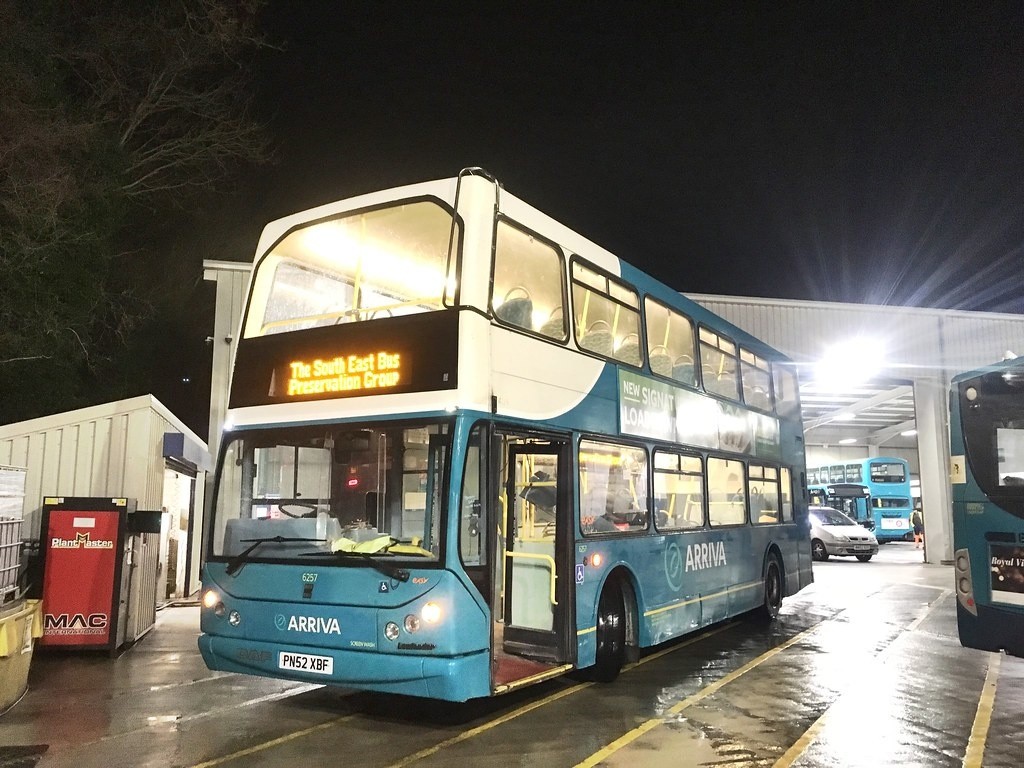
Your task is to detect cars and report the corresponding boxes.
[805,505,879,564]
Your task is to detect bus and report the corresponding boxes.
[807,482,876,540]
[805,456,915,544]
[948,357,1024,658]
[196,167,815,703]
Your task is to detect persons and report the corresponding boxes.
[910,509,924,548]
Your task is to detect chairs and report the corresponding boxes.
[687,518,723,527]
[498,285,795,419]
[335,309,397,322]
[806,476,862,484]
